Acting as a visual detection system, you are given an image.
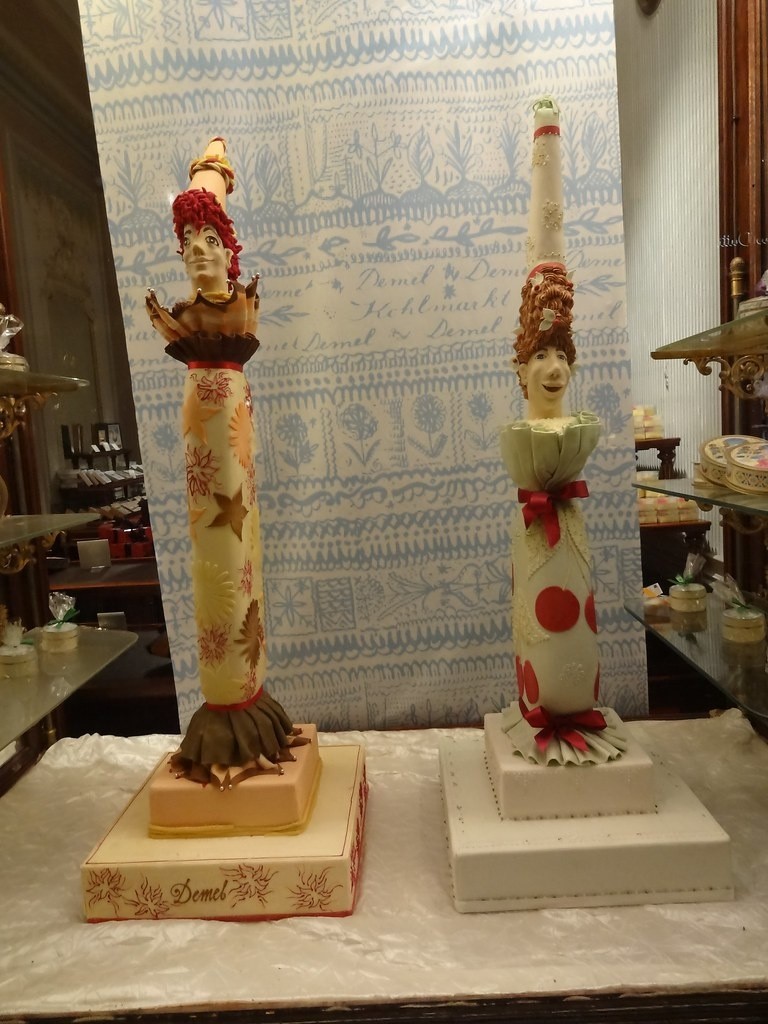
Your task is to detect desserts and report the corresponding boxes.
[631,403,699,524]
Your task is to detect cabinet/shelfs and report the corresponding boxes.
[0,367,143,753]
[628,310,768,721]
[61,450,146,509]
[635,437,714,553]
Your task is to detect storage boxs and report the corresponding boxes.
[66,495,153,560]
[63,478,84,483]
[131,464,138,469]
[93,469,112,485]
[116,470,133,481]
[137,465,143,472]
[80,470,94,487]
[105,470,125,481]
[60,483,79,488]
[126,469,144,479]
[88,469,102,485]
[60,473,78,478]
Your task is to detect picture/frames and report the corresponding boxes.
[91,422,109,451]
[106,421,123,450]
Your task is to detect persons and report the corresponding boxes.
[513,267,578,419]
[172,188,243,297]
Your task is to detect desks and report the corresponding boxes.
[45,563,164,624]
